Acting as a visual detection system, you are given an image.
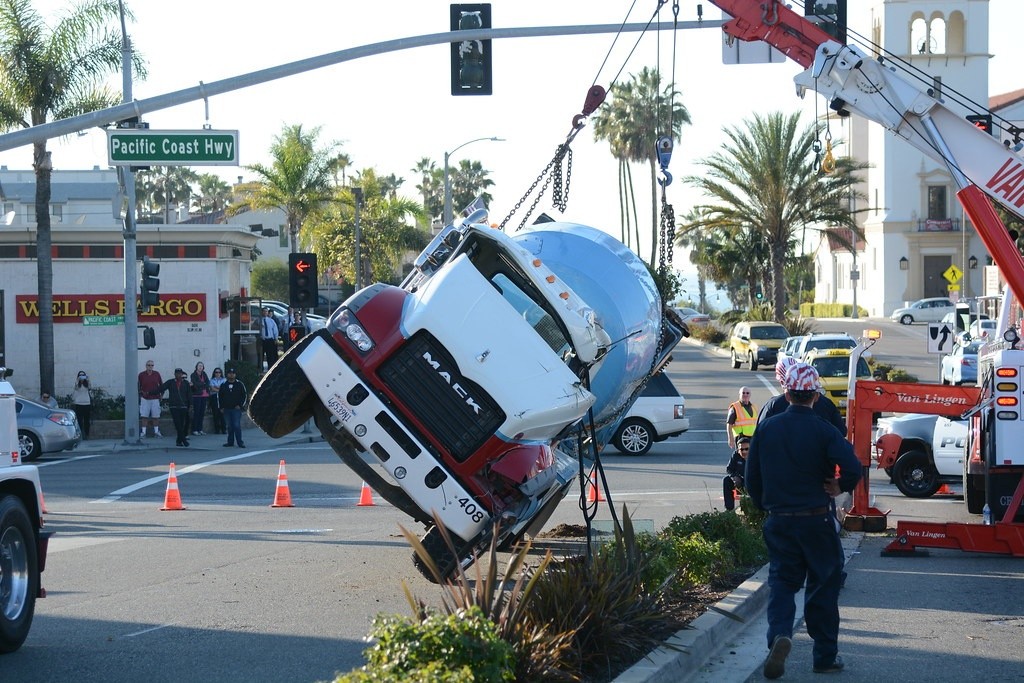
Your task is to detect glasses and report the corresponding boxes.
[148,364,154,367]
[42,397,50,399]
[79,374,85,377]
[215,372,221,374]
[739,447,749,451]
[741,391,751,394]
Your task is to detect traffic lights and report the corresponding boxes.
[755,283,763,300]
[144,327,156,350]
[289,325,305,346]
[288,253,319,308]
[140,256,160,312]
[965,114,994,136]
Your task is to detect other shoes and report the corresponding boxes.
[85,435,89,440]
[238,444,245,448]
[223,443,234,446]
[222,430,228,434]
[176,442,184,446]
[192,430,207,436]
[183,440,190,447]
[214,431,220,434]
[140,432,146,439]
[153,432,163,439]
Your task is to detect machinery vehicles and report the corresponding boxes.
[247,214,685,584]
[710,0,1024,559]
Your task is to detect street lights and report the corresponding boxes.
[350,187,362,295]
[443,136,506,227]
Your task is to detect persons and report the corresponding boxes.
[37,305,312,448]
[722,357,864,679]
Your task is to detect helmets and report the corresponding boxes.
[777,357,797,381]
[783,363,820,390]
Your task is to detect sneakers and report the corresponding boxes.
[813,655,845,671]
[764,637,792,678]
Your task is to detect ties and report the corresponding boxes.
[289,315,291,323]
[263,317,268,337]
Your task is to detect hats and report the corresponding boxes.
[175,368,182,373]
[228,369,237,373]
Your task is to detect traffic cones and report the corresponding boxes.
[159,461,187,511]
[589,471,607,502]
[356,479,375,506]
[269,459,295,507]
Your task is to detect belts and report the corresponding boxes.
[777,507,831,516]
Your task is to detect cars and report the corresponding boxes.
[240,295,343,338]
[14,397,83,463]
[940,339,981,388]
[775,330,878,417]
[939,312,998,342]
[606,369,688,457]
[673,308,711,328]
[891,297,955,325]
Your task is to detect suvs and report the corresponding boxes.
[0,366,57,656]
[729,321,791,372]
